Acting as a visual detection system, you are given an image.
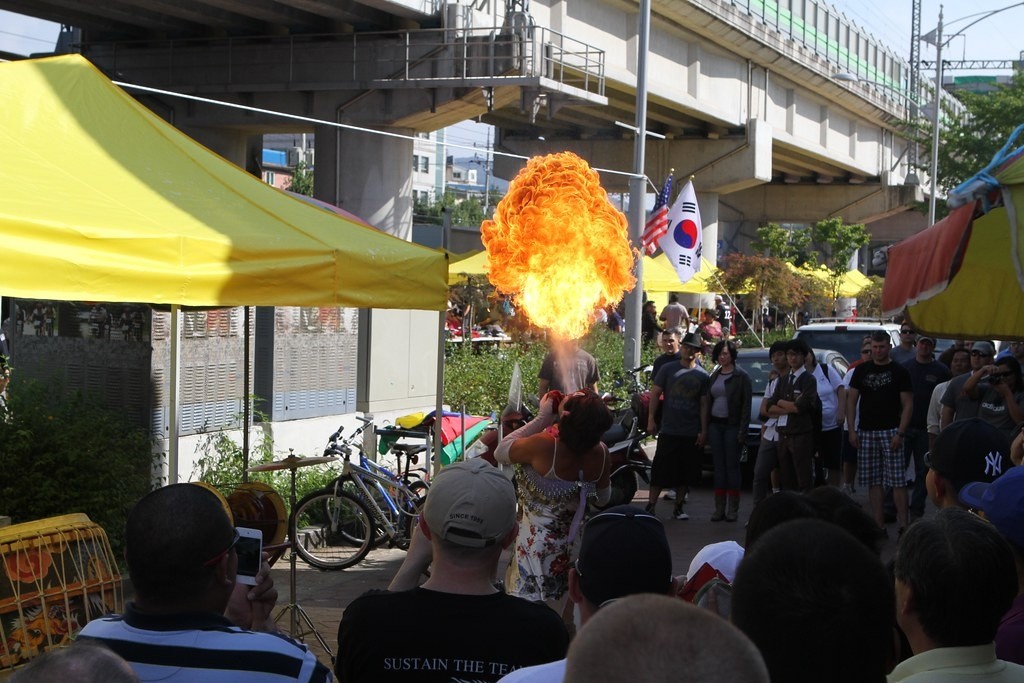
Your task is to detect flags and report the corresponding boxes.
[637,169,701,287]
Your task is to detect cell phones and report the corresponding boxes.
[234,526,262,585]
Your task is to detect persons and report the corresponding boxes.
[707,340,753,522]
[564,594,771,683]
[335,458,570,683]
[687,318,1024,666]
[493,388,613,603]
[886,507,1024,683]
[730,520,901,683]
[9,642,141,683]
[444,286,859,353]
[643,334,711,521]
[537,339,600,406]
[496,505,677,683]
[73,482,334,683]
[649,329,703,502]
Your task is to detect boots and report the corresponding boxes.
[727,496,739,522]
[711,495,726,522]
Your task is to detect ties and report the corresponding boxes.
[789,373,796,392]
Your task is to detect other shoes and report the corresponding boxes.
[841,484,852,499]
[665,488,690,503]
[674,509,689,520]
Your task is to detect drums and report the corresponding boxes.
[0,510,127,680]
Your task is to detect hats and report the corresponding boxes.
[575,504,672,608]
[971,341,993,356]
[917,335,937,347]
[679,333,704,350]
[686,539,744,586]
[423,457,518,548]
[924,417,1013,487]
[563,593,769,683]
[958,466,1024,547]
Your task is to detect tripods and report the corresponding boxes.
[243,455,334,662]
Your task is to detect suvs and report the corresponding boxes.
[791,316,904,364]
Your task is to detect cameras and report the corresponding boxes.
[990,372,1001,386]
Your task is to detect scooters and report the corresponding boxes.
[465,359,663,514]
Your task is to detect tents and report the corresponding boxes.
[879,147,1024,340]
[450,250,885,298]
[0,54,449,419]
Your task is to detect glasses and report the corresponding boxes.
[861,349,872,353]
[901,329,915,335]
[972,350,989,357]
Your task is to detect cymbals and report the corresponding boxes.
[245,456,339,473]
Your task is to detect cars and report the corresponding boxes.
[707,346,850,451]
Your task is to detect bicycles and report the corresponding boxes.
[288,415,432,578]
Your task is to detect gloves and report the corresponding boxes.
[493,393,553,466]
